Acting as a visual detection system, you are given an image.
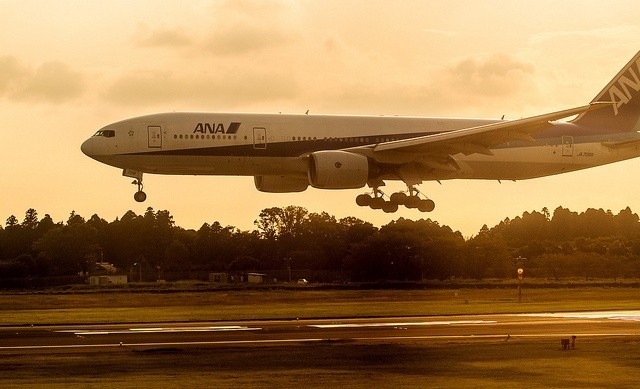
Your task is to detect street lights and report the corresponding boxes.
[517,268,524,303]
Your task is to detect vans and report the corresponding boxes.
[298,278,309,285]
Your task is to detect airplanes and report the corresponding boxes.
[79,46,640,216]
